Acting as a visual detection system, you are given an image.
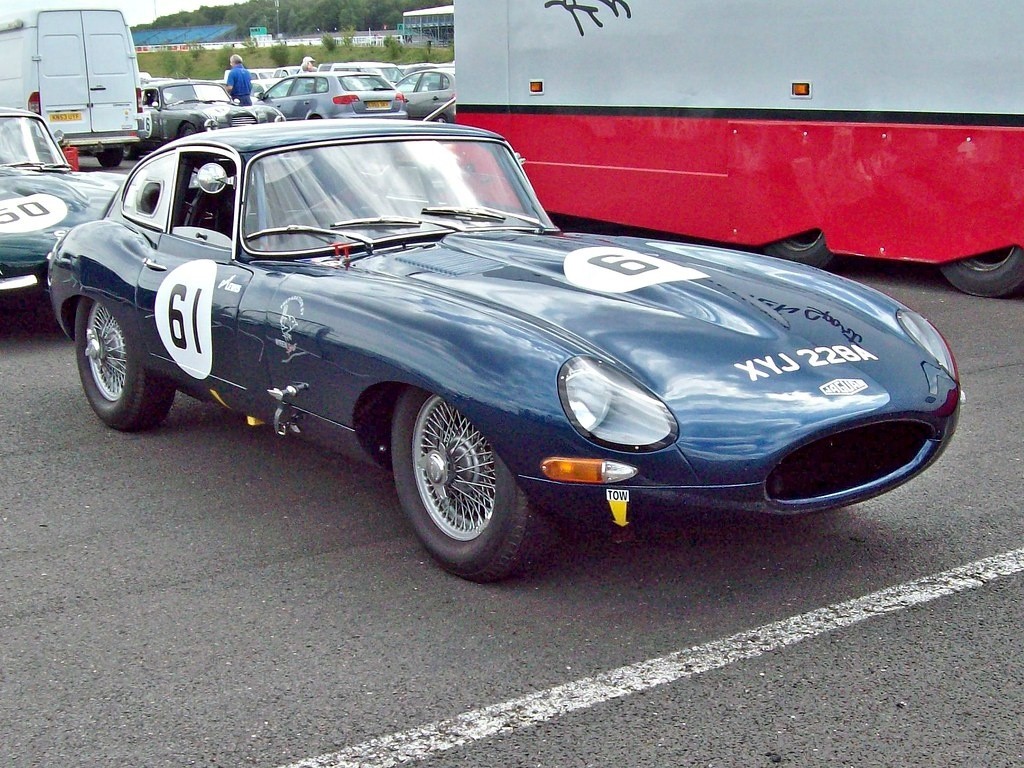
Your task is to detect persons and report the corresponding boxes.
[301,55,317,71]
[225,54,252,106]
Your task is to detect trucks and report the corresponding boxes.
[0,7,152,166]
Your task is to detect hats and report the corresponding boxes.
[303,57,315,62]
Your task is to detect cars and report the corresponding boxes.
[47,118,966,582]
[123,61,455,160]
[0,106,119,290]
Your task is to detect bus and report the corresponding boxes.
[456,0,1024,297]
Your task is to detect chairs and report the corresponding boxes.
[150,183,237,241]
